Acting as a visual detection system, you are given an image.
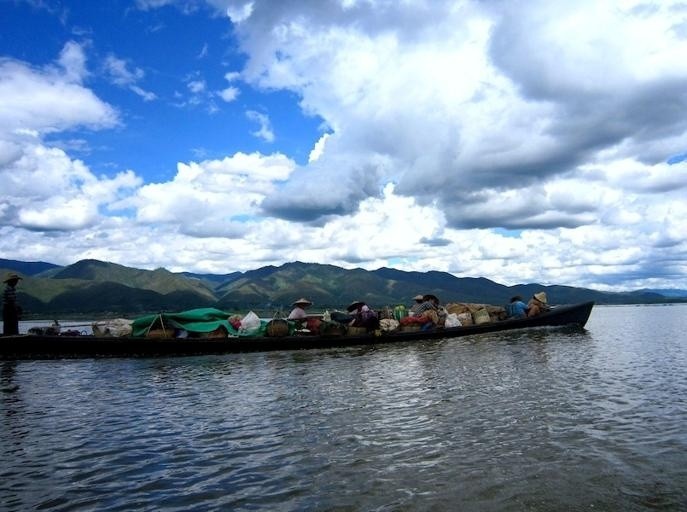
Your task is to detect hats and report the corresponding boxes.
[346,301,365,310]
[3,272,23,282]
[510,296,523,303]
[412,294,440,304]
[294,298,312,304]
[534,292,547,304]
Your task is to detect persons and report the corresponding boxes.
[347,301,360,315]
[2,273,23,334]
[410,294,444,329]
[287,298,312,321]
[507,291,552,318]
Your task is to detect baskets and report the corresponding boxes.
[144,329,175,337]
[472,309,490,324]
[325,325,343,336]
[266,319,288,336]
[346,327,368,337]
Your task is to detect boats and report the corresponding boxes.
[0,297,598,360]
